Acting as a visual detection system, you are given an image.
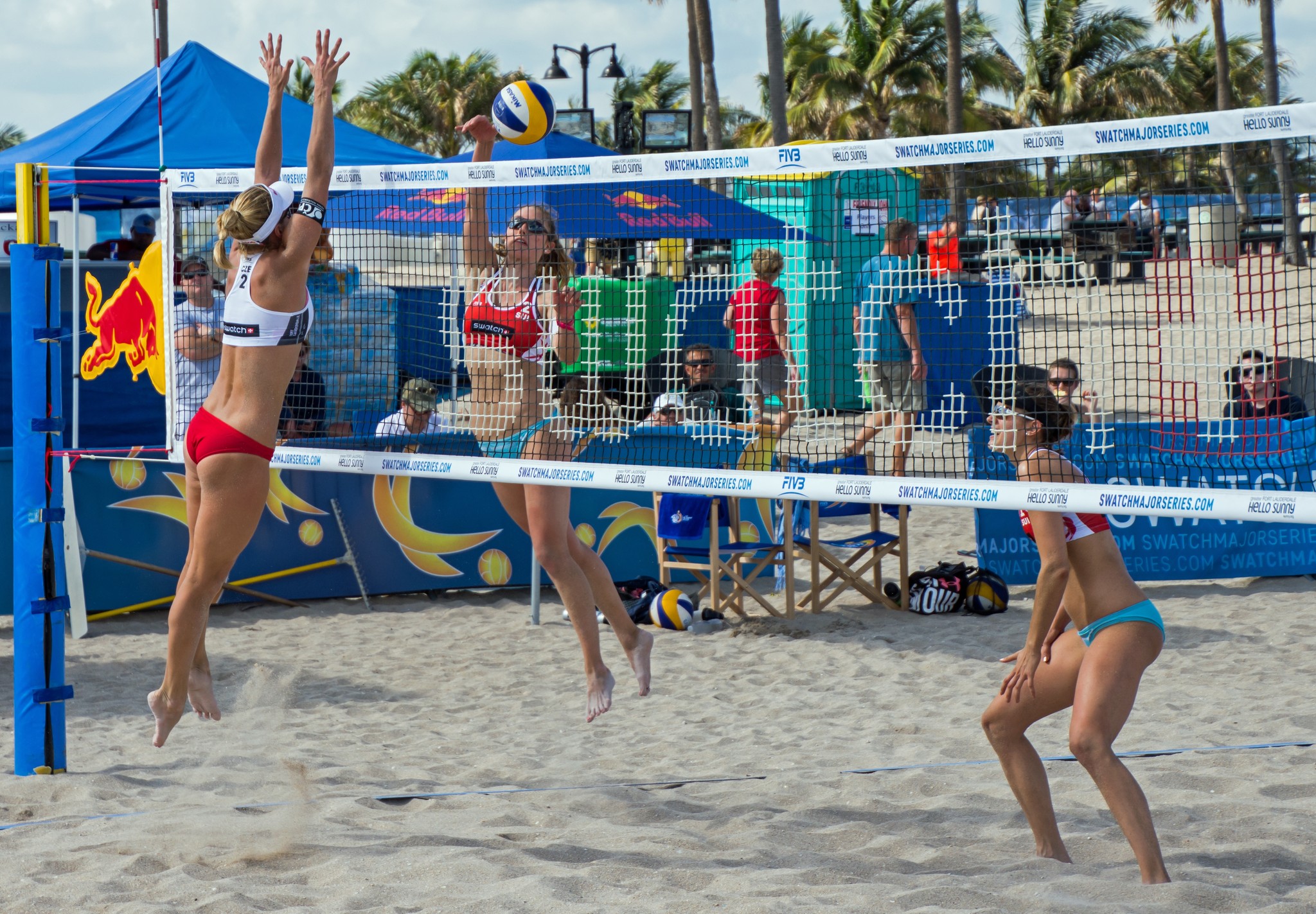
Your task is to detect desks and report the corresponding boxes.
[919,227,1075,278]
[1073,217,1168,281]
[61,424,778,613]
[1165,211,1316,261]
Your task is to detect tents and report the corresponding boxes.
[0,40,442,447]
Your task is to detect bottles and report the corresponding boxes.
[562,608,609,624]
[1117,419,1123,423]
[884,582,901,607]
[687,607,726,633]
[749,400,761,424]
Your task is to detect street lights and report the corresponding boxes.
[540,43,627,108]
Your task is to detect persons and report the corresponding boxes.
[1122,188,1161,258]
[1047,188,1110,233]
[926,214,970,281]
[1223,349,1309,421]
[981,380,1171,885]
[1046,358,1102,424]
[454,115,653,722]
[170,238,806,454]
[87,214,157,261]
[971,195,1001,233]
[836,218,928,477]
[149,28,351,748]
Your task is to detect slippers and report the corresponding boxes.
[957,549,977,557]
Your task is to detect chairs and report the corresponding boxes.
[653,463,795,619]
[782,451,910,615]
[970,364,1049,426]
[352,409,401,437]
[643,345,745,408]
[1223,356,1316,417]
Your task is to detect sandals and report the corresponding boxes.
[836,446,854,459]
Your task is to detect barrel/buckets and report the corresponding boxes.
[1189,203,1240,267]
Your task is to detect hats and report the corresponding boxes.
[1138,187,1150,197]
[236,181,294,246]
[402,377,438,412]
[983,384,1062,436]
[132,213,156,236]
[654,393,683,415]
[1299,193,1309,197]
[181,255,209,276]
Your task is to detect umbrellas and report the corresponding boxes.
[318,130,833,245]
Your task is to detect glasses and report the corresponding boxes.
[406,402,437,416]
[686,359,714,367]
[181,269,211,280]
[1048,378,1076,387]
[508,216,551,242]
[278,207,292,222]
[415,386,440,398]
[660,406,683,415]
[994,405,1046,426]
[1238,364,1272,377]
[299,350,306,357]
[909,235,918,246]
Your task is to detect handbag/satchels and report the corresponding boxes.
[613,575,669,625]
[909,560,977,615]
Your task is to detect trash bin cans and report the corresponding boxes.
[1188,204,1240,267]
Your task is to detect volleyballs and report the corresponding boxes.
[966,574,1009,613]
[491,80,557,145]
[649,588,693,631]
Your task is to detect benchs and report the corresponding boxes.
[919,229,1316,288]
